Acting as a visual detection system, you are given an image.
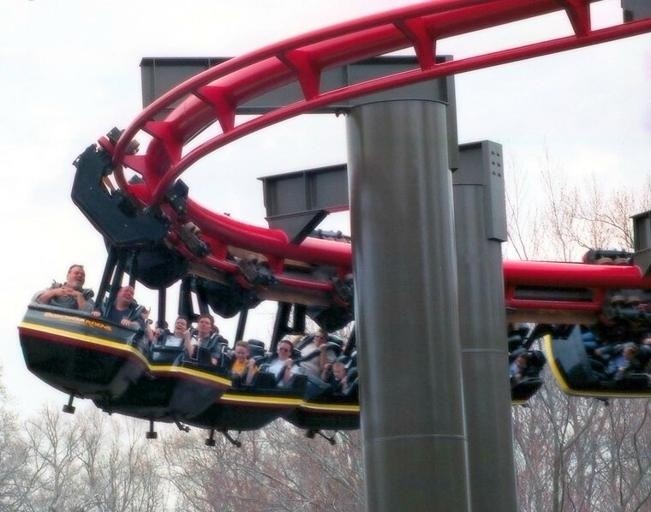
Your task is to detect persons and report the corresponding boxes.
[232,331,349,392]
[509,354,528,380]
[155,313,221,366]
[33,264,95,314]
[582,302,651,381]
[92,286,145,330]
